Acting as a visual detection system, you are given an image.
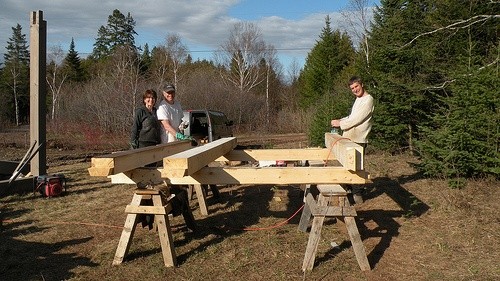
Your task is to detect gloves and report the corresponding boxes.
[175,131,196,140]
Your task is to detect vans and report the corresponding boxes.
[187,109,235,145]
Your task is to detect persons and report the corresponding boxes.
[130,89,163,168]
[156,83,199,145]
[331,77,376,171]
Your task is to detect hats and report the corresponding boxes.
[164,82,176,92]
